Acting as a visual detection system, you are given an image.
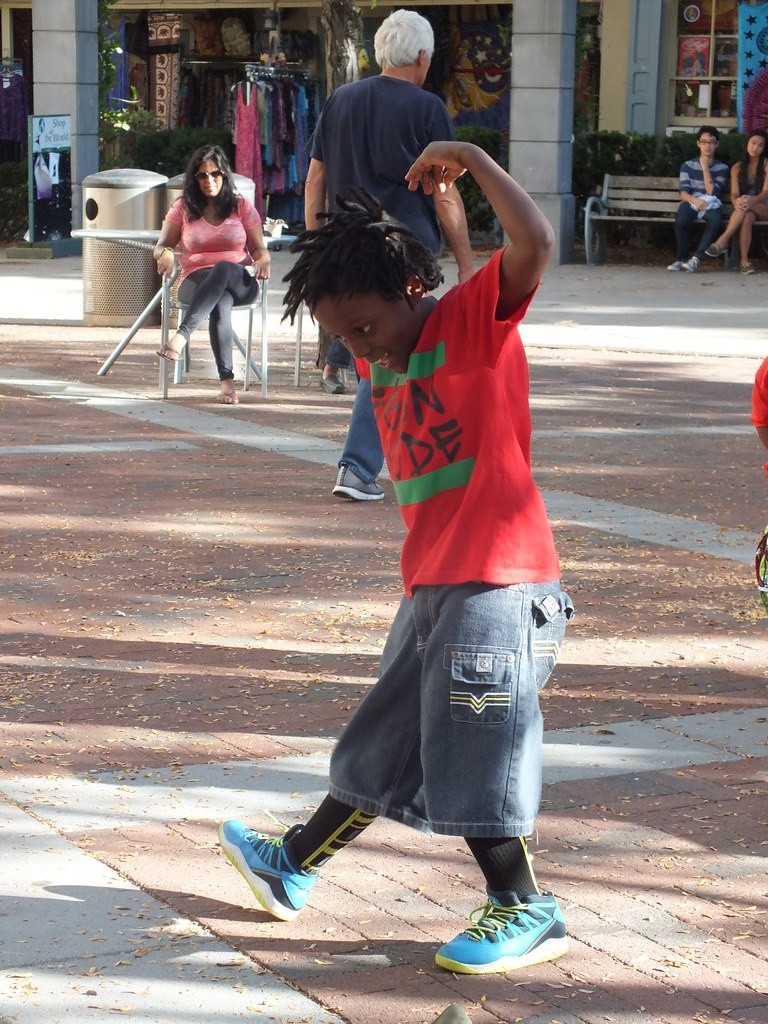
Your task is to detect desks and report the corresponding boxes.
[71,228,308,380]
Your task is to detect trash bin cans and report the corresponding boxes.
[166,171,255,330]
[82,168,168,326]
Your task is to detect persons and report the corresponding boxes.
[153,144,271,404]
[303,7,476,502]
[666,124,729,272]
[215,142,578,975]
[318,335,361,394]
[705,128,768,275]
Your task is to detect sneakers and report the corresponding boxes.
[435,884,567,974]
[331,464,384,501]
[219,813,319,922]
[667,261,684,271]
[683,257,700,273]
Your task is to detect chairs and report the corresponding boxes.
[161,245,268,399]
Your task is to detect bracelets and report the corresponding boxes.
[161,247,174,257]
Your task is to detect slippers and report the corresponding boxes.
[217,389,239,405]
[158,346,185,363]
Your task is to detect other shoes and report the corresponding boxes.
[318,376,345,394]
[705,244,727,257]
[739,261,754,274]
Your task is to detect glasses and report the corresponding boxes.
[196,170,226,181]
[697,139,717,146]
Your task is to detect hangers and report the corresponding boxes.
[230,63,321,88]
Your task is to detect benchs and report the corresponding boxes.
[582,172,768,270]
